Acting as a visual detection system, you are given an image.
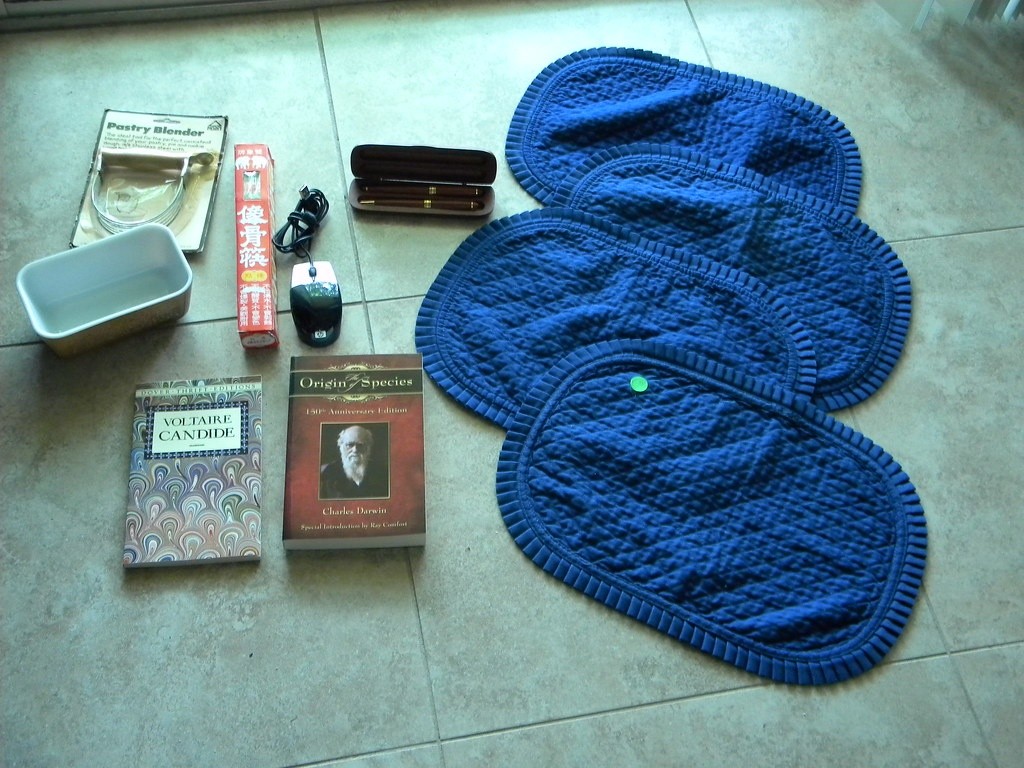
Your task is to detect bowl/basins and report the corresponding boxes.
[15,222,193,357]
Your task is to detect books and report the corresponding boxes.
[282,353,427,550]
[122,374,263,568]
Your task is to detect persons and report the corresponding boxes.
[320,424,388,499]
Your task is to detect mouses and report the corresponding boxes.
[290,261,343,347]
